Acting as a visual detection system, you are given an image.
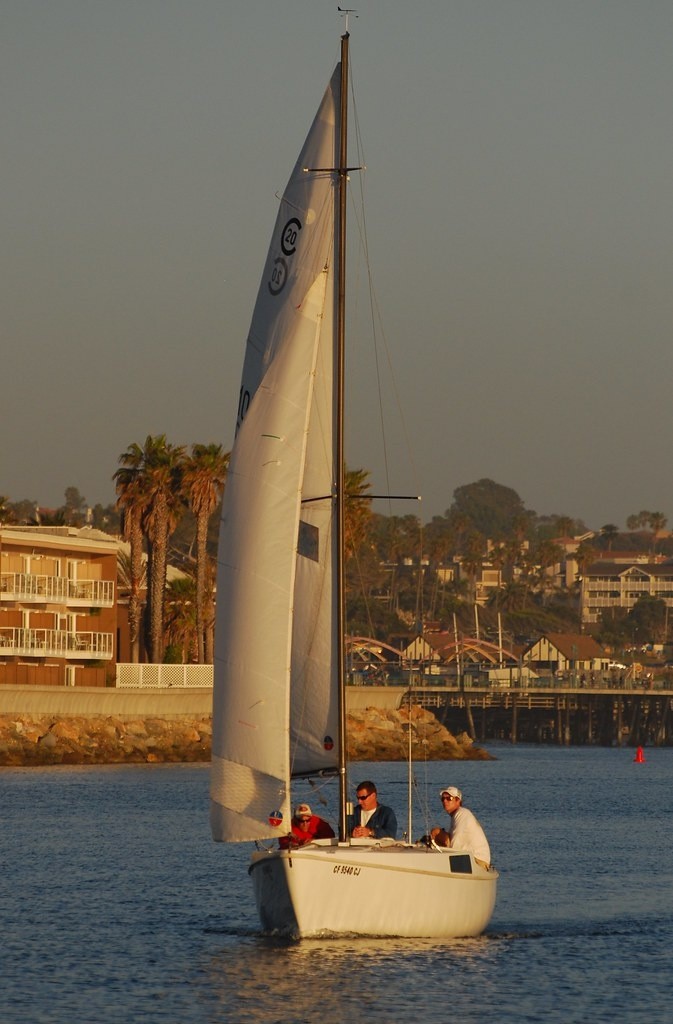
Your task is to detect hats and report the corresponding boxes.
[294,803,311,816]
[439,786,462,799]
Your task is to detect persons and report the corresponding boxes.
[276,803,336,849]
[342,777,398,842]
[428,785,494,874]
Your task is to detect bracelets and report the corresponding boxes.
[367,827,374,837]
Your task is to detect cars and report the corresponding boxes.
[610,661,626,669]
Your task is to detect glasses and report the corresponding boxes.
[440,796,459,801]
[356,793,372,800]
[297,818,311,823]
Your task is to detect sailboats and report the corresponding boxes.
[208,6,499,941]
